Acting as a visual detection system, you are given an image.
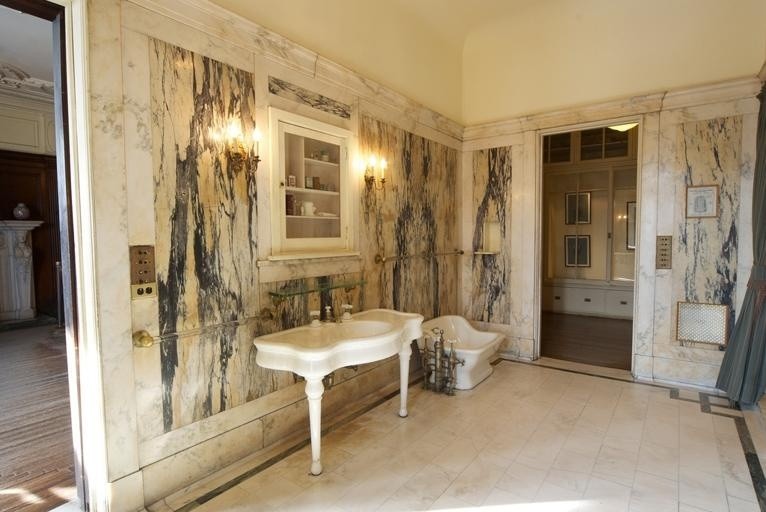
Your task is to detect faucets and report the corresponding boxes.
[323,305,343,324]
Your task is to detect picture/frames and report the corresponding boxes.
[563,192,591,268]
[684,185,720,220]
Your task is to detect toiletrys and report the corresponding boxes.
[285,147,337,216]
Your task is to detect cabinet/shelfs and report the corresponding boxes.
[265,107,358,262]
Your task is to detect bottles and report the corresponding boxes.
[288,174,297,187]
[309,150,331,162]
[306,176,320,189]
[286,193,314,217]
[13,203,30,220]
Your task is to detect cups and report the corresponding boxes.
[320,184,336,191]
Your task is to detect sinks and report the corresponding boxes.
[418,314,505,391]
[252,307,424,375]
